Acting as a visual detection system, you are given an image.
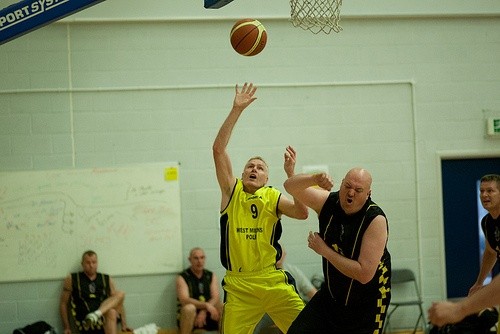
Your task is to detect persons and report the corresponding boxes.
[252,241,318,334]
[426,274,500,334]
[213,81,309,334]
[176,247,223,334]
[469,174,500,296]
[283,167,391,334]
[59,251,133,334]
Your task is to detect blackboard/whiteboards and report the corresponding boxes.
[0,160,185,284]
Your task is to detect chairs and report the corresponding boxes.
[380,269,427,334]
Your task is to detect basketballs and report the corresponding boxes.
[230,18,268,56]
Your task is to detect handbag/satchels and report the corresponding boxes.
[13,321,57,334]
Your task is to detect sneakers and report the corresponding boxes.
[84,313,100,327]
